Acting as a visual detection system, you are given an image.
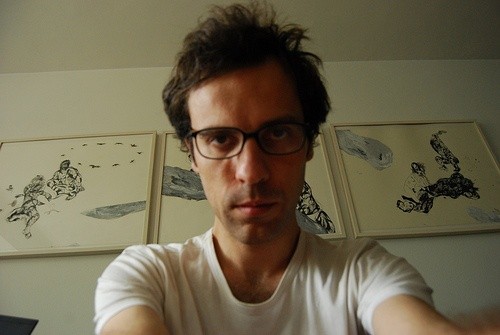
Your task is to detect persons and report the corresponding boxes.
[93,0,473,335]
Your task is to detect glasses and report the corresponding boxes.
[188,122,307,160]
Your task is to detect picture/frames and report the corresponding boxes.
[0,130,157,258]
[153,131,347,244]
[330,118,500,239]
